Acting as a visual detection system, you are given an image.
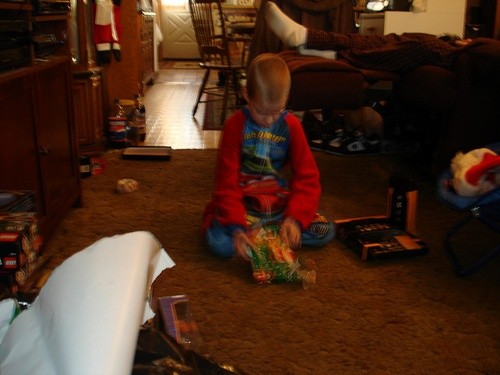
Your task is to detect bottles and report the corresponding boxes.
[107,98,128,149]
[128,94,147,146]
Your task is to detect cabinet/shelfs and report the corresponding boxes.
[0,0,163,235]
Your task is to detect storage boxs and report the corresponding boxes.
[122,146,171,160]
[332,179,429,263]
[0,190,43,301]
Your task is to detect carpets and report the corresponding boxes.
[40,147,500,375]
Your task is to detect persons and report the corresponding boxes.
[263,0,500,63]
[198,54,339,259]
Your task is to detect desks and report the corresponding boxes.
[337,39,458,159]
[217,20,255,64]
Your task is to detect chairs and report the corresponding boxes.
[189,0,254,125]
